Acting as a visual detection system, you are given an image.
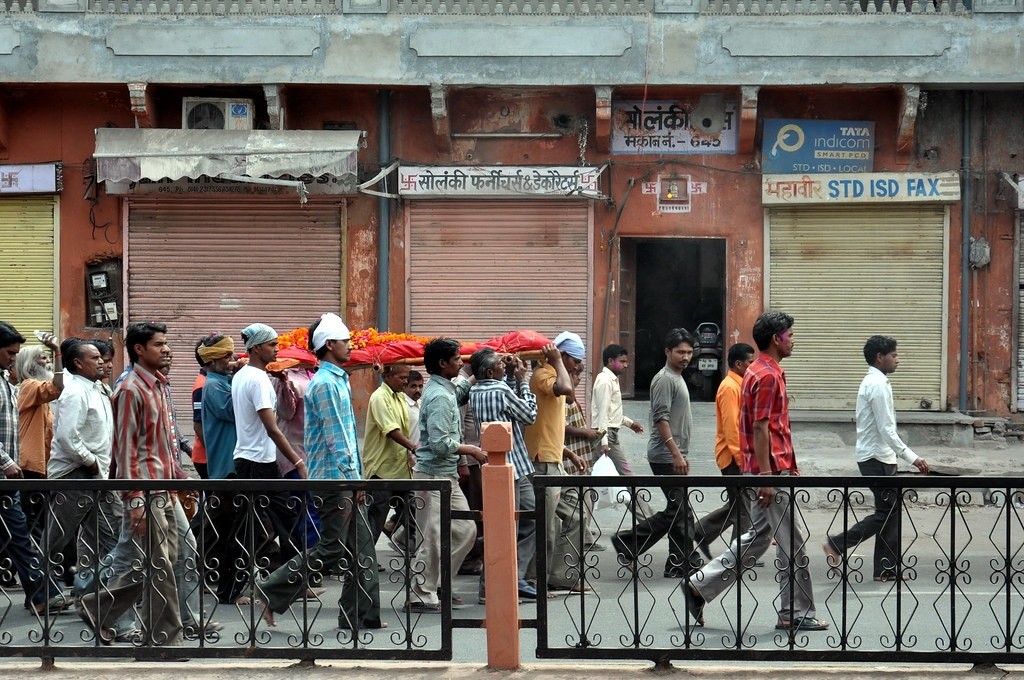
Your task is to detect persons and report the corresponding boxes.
[0,321,225,661]
[192,313,608,630]
[681,313,829,630]
[611,329,697,578]
[823,335,929,580]
[592,344,653,523]
[694,342,765,566]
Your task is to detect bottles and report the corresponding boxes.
[34,331,57,343]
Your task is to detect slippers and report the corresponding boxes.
[382,526,393,541]
[681,580,706,627]
[34,597,73,617]
[775,617,830,630]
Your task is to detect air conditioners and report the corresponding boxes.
[181,96,255,131]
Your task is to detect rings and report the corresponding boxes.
[135,523,140,527]
[760,496,764,499]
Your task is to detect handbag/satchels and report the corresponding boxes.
[591,448,632,510]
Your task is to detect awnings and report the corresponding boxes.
[93,128,368,182]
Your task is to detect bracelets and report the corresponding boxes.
[759,471,772,474]
[295,459,302,466]
[665,437,672,444]
[54,372,64,374]
[55,353,62,357]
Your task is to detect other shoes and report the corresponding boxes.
[79,596,114,645]
[756,560,763,566]
[584,542,607,551]
[693,523,713,560]
[115,629,142,643]
[479,596,522,604]
[387,541,415,558]
[611,535,633,570]
[664,569,698,578]
[403,601,442,614]
[136,657,190,662]
[183,617,224,635]
[516,578,538,599]
[458,563,484,575]
[436,586,464,606]
[0,584,24,591]
